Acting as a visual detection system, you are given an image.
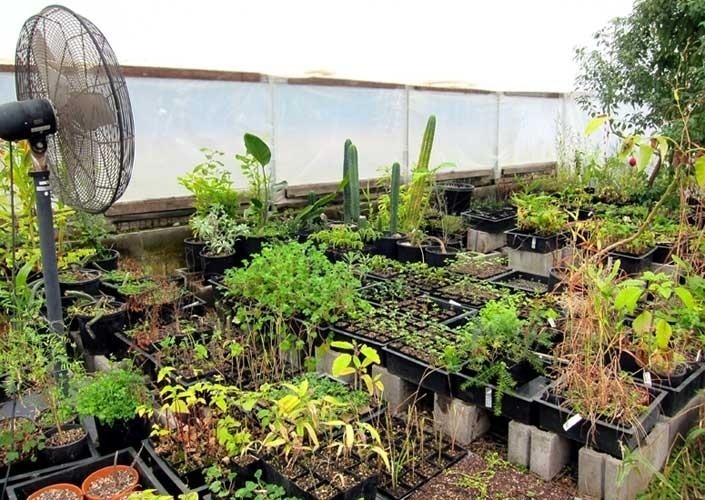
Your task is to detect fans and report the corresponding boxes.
[0,5,135,369]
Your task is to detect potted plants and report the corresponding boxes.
[0,116,705,500]
